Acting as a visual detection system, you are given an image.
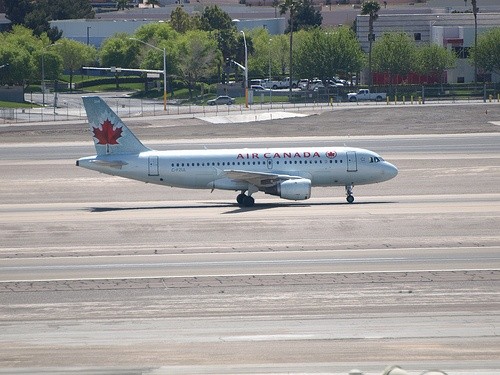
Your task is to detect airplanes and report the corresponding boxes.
[74,95,398,207]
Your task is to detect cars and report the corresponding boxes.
[219,77,356,91]
[207,96,236,105]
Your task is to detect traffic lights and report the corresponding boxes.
[226,57,231,66]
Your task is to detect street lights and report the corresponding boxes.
[240,30,249,107]
[268,39,273,103]
[129,37,168,110]
[42,42,62,107]
[87,26,93,79]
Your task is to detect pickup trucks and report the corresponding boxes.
[347,88,388,102]
